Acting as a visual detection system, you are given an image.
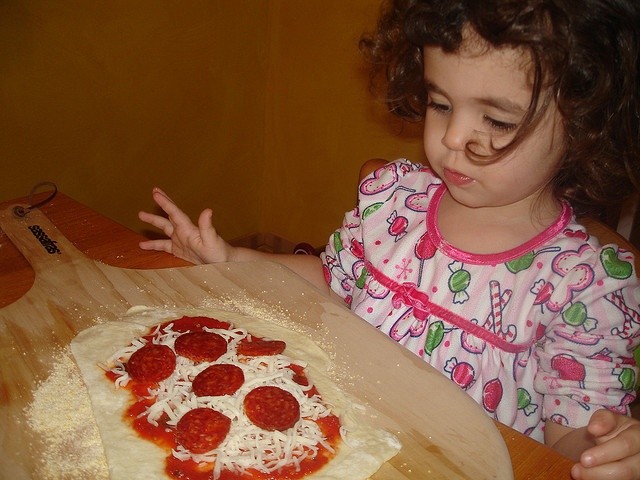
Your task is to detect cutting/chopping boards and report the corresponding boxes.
[0,203,514,480]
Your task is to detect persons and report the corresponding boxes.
[135,1,639,478]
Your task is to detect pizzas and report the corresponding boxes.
[70,305,402,476]
